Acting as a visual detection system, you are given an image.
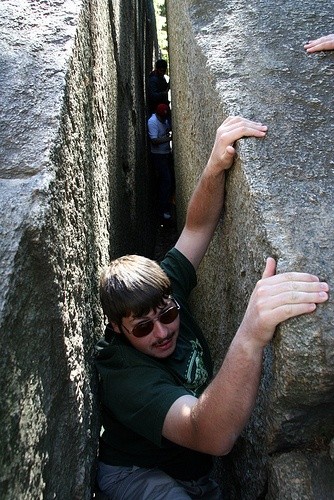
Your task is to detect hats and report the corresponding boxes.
[156,103,169,113]
[156,59,167,70]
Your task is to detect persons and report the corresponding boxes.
[96,116,329,500]
[148,59,170,115]
[147,104,175,219]
[304,33,334,52]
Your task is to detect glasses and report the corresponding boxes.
[115,294,181,338]
